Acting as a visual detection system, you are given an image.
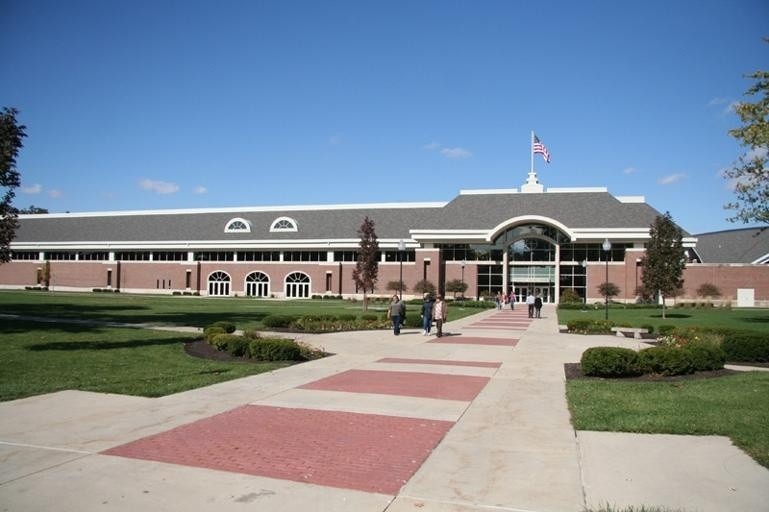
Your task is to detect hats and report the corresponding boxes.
[424,295,432,300]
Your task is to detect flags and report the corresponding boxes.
[534,136,551,163]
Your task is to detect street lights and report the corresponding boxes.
[602,237,611,321]
[460,258,465,302]
[398,239,406,300]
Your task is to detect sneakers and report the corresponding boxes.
[428,333,430,336]
[423,330,426,336]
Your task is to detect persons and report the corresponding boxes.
[534,295,542,318]
[420,293,434,336]
[509,291,516,310]
[501,293,505,309]
[387,295,404,335]
[431,295,448,337]
[526,294,535,318]
[496,291,501,310]
